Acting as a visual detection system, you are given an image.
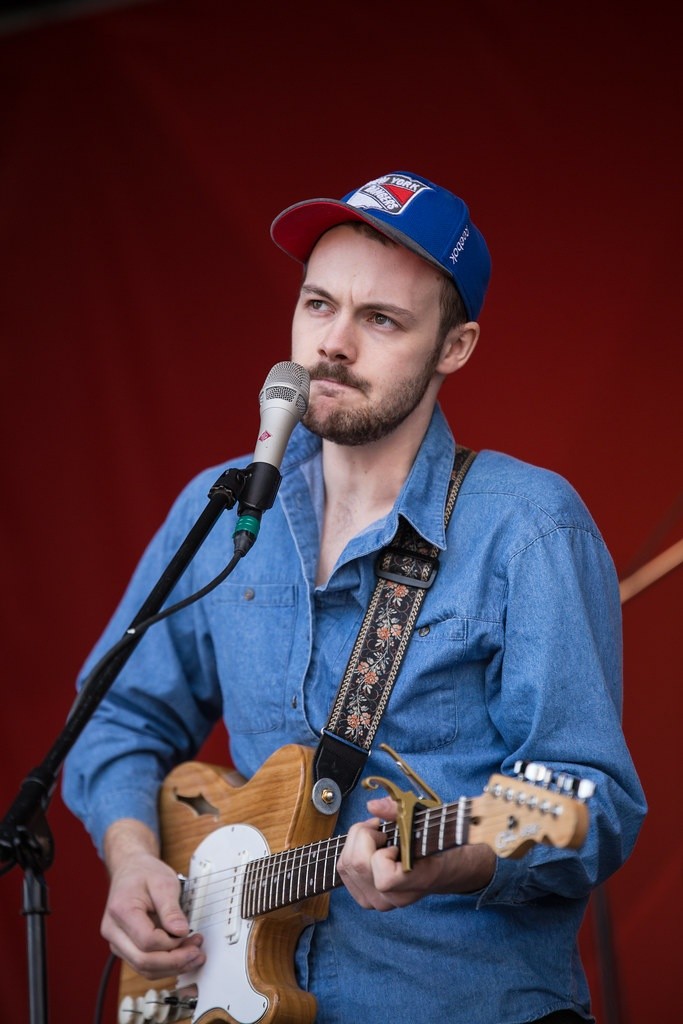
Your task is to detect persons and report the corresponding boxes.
[61,170,648,1024]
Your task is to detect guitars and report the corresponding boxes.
[117,743,595,1024]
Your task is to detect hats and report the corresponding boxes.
[270,170,490,323]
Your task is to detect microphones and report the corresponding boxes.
[231,362,310,558]
